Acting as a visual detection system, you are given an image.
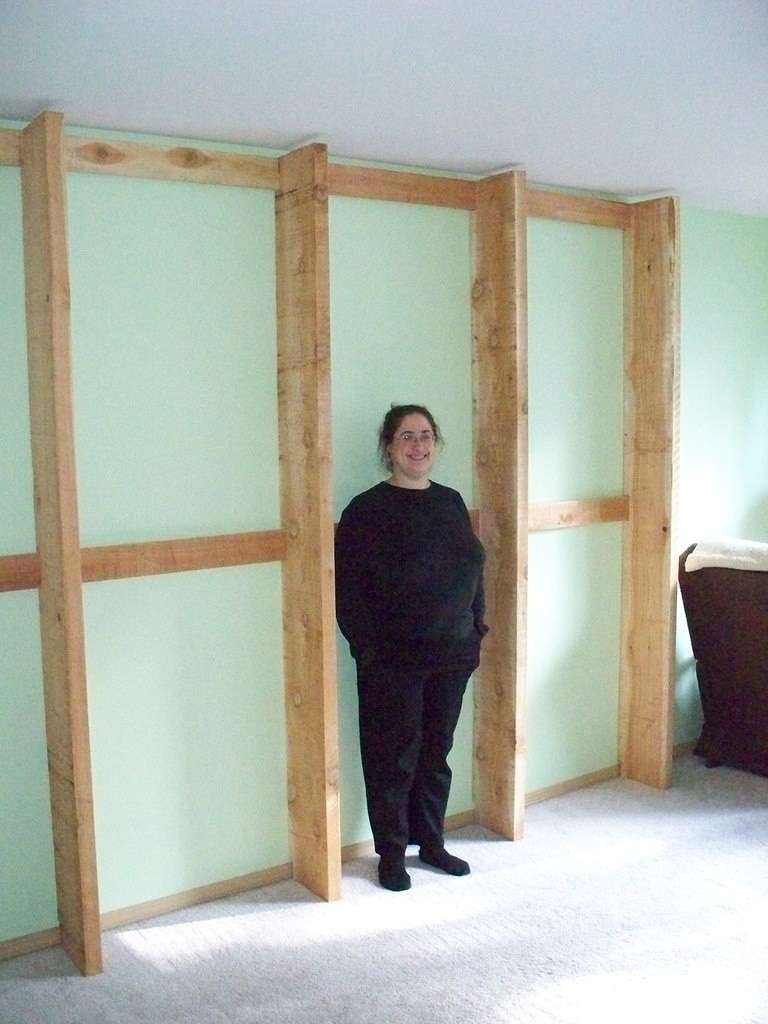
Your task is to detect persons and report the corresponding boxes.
[334,403,489,892]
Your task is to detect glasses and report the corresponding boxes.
[393,434,436,446]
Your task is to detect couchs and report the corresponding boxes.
[676,535,768,782]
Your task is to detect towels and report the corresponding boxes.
[683,537,768,572]
